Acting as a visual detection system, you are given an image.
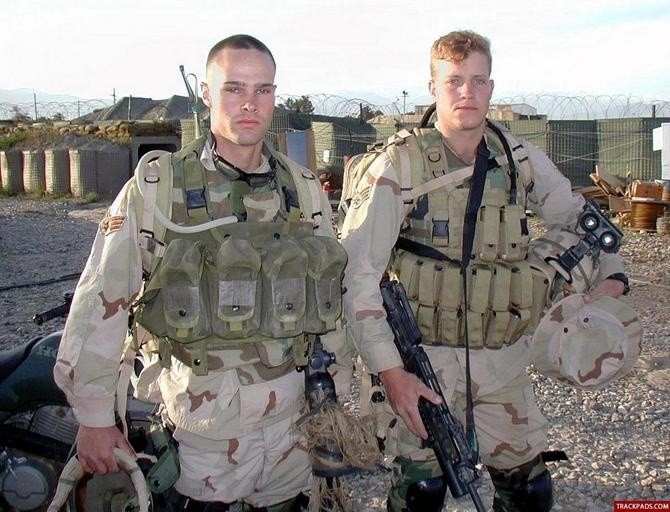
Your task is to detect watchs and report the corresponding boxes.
[608,273,630,295]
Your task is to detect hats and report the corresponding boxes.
[531,293,643,391]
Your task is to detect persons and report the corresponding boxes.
[53,34,358,511]
[340,30,631,511]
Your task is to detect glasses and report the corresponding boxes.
[212,151,278,187]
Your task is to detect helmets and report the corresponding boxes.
[529,228,600,303]
[47,447,155,512]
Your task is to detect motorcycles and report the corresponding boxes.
[0,286,180,512]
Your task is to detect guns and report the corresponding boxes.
[380,272,483,511]
